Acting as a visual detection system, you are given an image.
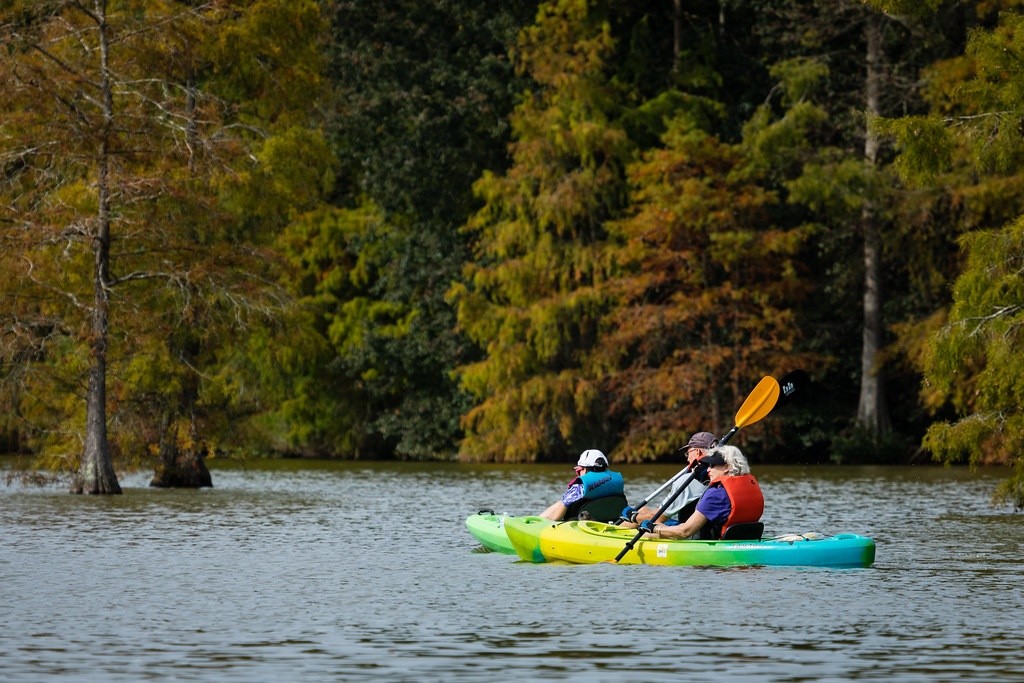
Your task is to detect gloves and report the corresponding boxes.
[619,506,639,523]
[637,520,657,533]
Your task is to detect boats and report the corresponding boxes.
[464,509,562,557]
[503,513,876,570]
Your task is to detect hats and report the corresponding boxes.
[698,452,726,464]
[678,432,719,451]
[573,449,609,469]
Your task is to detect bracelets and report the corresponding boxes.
[653,525,664,538]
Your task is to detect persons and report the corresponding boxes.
[618,432,720,529]
[637,446,765,540]
[537,449,623,521]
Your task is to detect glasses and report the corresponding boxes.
[709,463,717,468]
[684,449,695,457]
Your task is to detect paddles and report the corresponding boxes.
[612,460,703,564]
[615,375,780,528]
